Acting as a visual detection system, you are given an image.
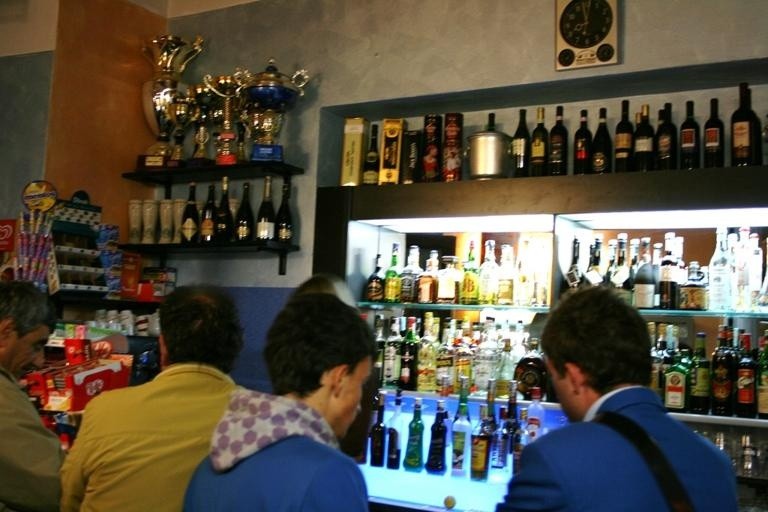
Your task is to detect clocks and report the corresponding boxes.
[555,0,618,71]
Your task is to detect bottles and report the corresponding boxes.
[385,243,401,302]
[507,379,518,455]
[658,109,664,127]
[680,100,700,168]
[402,245,423,301]
[550,106,568,175]
[615,100,634,172]
[634,104,655,172]
[196,200,205,215]
[649,316,768,422]
[215,177,233,240]
[635,111,642,128]
[256,176,276,241]
[386,398,406,469]
[416,259,438,303]
[200,185,218,241]
[143,199,157,244]
[276,176,294,243]
[369,395,386,467]
[365,253,385,301]
[490,406,506,468]
[174,199,186,243]
[517,407,530,453]
[685,260,709,309]
[360,311,545,399]
[656,103,677,169]
[486,112,497,131]
[500,242,532,305]
[453,375,472,425]
[437,257,463,298]
[530,386,544,443]
[451,401,470,477]
[470,403,493,481]
[158,199,173,244]
[425,400,448,475]
[709,225,764,312]
[441,376,452,447]
[128,199,142,243]
[180,182,199,244]
[562,230,685,308]
[229,199,240,215]
[532,106,548,176]
[704,98,725,166]
[361,124,380,184]
[402,398,424,472]
[234,182,255,242]
[513,108,531,175]
[479,239,499,303]
[573,109,593,174]
[593,107,612,173]
[485,378,496,431]
[460,240,479,305]
[730,82,763,166]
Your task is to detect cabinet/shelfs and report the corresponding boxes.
[313,164,768,485]
[45,291,162,358]
[121,160,303,275]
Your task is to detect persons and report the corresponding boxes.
[494,284,739,512]
[287,274,377,463]
[0,279,68,512]
[183,294,378,512]
[59,285,246,512]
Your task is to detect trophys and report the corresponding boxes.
[136,35,313,170]
